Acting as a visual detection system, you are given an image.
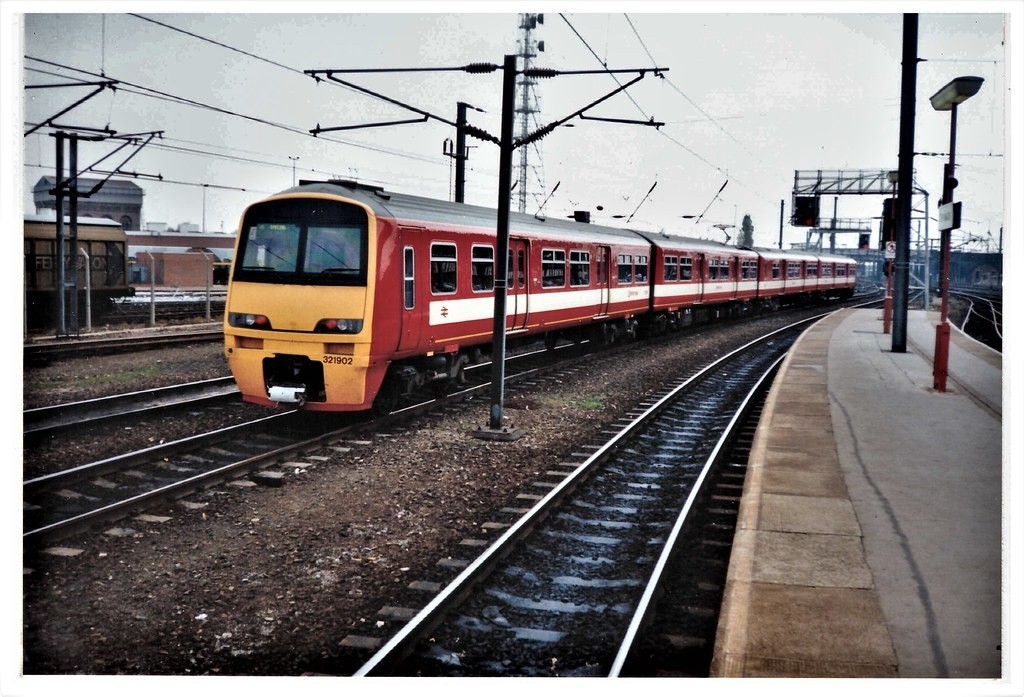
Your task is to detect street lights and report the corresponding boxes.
[289,156,299,186]
[883,169,898,335]
[928,75,985,394]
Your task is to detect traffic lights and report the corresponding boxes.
[859,233,870,249]
[792,196,820,228]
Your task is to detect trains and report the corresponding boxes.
[24,213,135,329]
[223,178,857,413]
